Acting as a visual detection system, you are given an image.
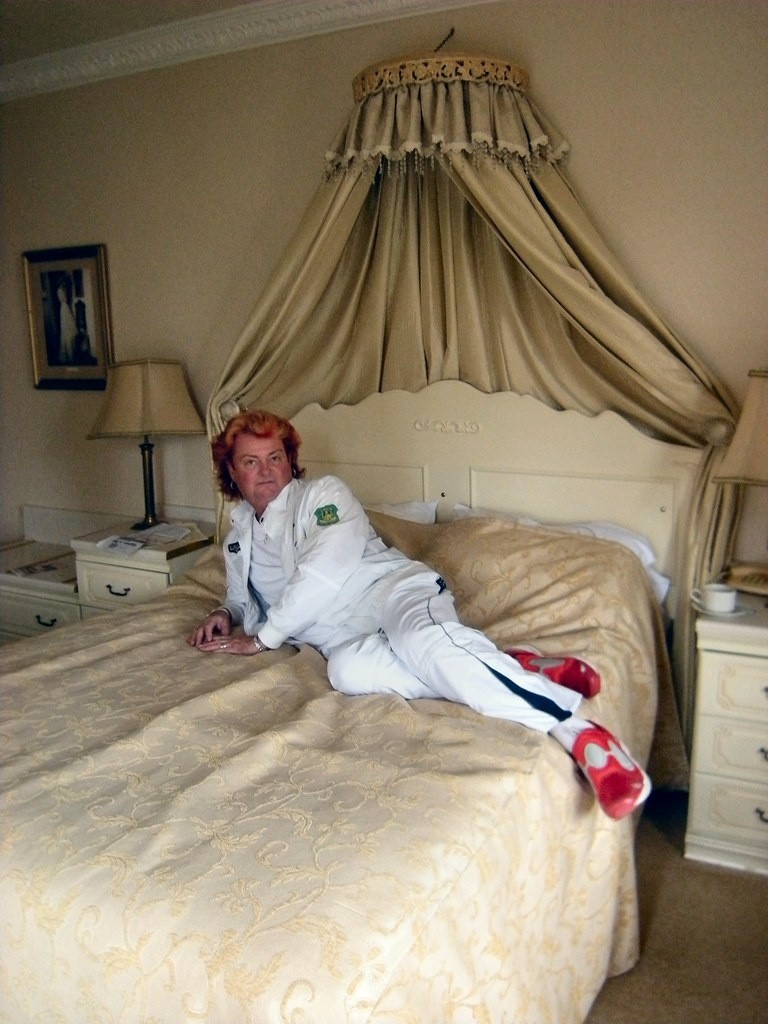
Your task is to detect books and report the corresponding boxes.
[125,522,191,542]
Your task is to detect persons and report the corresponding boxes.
[186,411,653,821]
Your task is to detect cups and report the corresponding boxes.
[688,584,737,612]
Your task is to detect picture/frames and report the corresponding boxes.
[21,243,115,391]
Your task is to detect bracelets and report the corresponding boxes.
[254,633,266,652]
[206,607,230,618]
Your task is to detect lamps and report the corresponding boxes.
[711,370,768,486]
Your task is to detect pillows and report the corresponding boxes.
[363,499,442,523]
[454,503,670,605]
[87,357,208,529]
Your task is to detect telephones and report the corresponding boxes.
[727,562,768,596]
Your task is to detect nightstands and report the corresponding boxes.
[685,590,768,875]
[1,523,216,647]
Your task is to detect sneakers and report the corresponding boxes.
[570,719,652,820]
[505,644,601,699]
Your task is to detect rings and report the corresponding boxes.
[193,629,196,631]
[221,643,226,649]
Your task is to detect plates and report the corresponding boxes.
[691,601,750,618]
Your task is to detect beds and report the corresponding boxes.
[0,380,692,1023]
